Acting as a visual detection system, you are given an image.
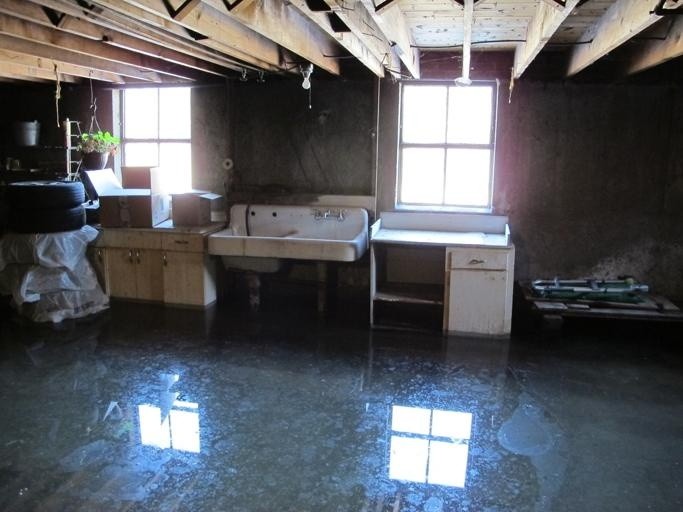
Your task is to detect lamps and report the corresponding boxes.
[300,62,314,90]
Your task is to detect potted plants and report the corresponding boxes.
[80,130,120,170]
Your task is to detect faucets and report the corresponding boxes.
[335,207,346,220]
[324,209,331,218]
[312,207,322,220]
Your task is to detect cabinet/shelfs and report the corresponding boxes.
[369,211,515,338]
[94,219,225,306]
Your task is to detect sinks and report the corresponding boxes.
[208,228,296,258]
[283,231,367,262]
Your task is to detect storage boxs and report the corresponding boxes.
[83,166,171,227]
[170,189,223,227]
